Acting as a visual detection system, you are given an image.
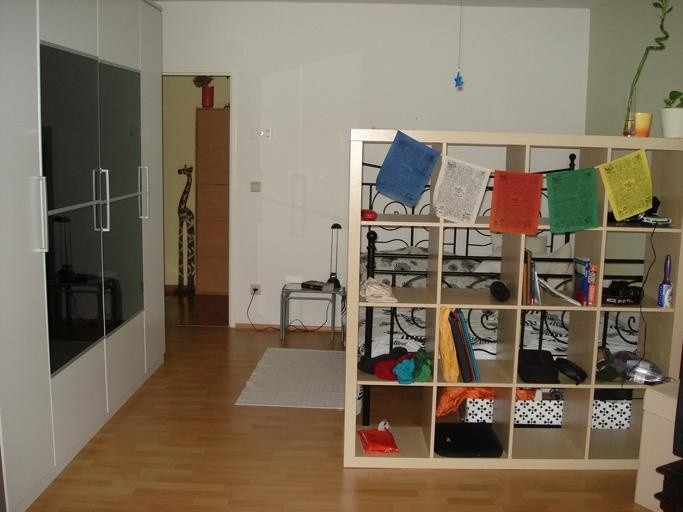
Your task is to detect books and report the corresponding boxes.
[522,247,541,305]
[572,255,591,307]
[585,265,596,307]
[448,308,480,383]
[537,276,581,309]
[300,280,335,291]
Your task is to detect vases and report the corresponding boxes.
[627,111,653,138]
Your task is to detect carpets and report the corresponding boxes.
[234,346,346,410]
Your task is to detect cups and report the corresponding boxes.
[625,112,652,136]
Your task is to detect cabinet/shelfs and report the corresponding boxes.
[343,128,683,470]
[0,0,168,512]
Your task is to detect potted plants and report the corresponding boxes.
[659,90,683,138]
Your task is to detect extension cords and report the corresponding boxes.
[322,281,334,294]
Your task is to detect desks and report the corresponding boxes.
[279,282,341,342]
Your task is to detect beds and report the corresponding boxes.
[341,154,644,427]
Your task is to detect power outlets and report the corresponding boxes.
[249,283,262,295]
